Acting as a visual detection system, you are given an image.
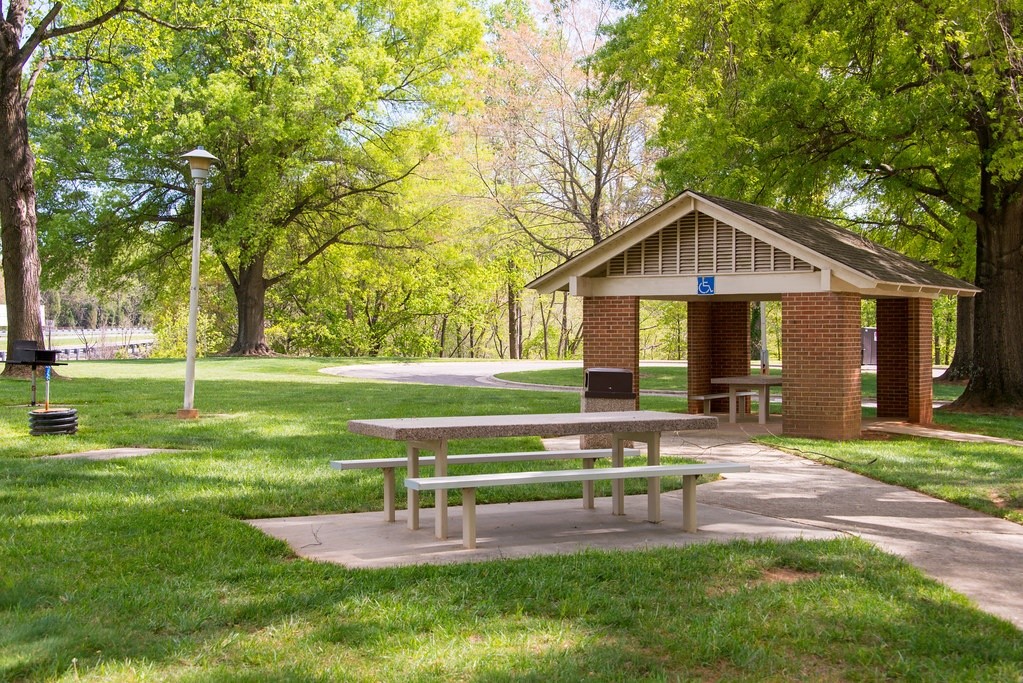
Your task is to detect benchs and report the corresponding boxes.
[328,447,641,522]
[404,462,749,550]
[690,391,759,415]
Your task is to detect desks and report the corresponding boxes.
[345,410,719,540]
[711,375,782,424]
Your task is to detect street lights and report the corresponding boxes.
[177,146,221,419]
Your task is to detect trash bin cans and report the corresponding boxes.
[579,366,637,451]
